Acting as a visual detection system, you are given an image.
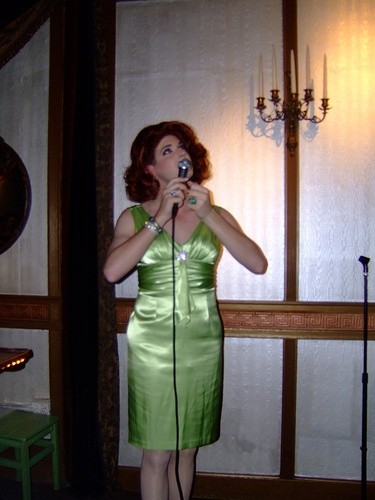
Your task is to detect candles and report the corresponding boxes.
[323,52,328,98]
[306,44,311,89]
[290,49,297,93]
[272,45,278,90]
[259,49,264,97]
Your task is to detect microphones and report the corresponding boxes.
[172,159,192,216]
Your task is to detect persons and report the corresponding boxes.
[104,119,269,500]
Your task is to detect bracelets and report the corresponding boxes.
[143,216,163,235]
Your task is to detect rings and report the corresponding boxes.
[170,189,177,198]
[187,196,198,204]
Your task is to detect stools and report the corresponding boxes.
[0,409,61,500]
